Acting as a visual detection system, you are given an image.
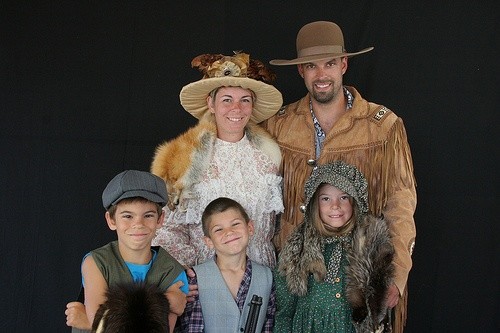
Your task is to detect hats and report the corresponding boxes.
[102,170,169,210]
[304,159,369,228]
[179,49,284,125]
[269,21,375,66]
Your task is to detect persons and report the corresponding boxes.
[272,160,394,333]
[65,170,189,333]
[151,53,285,304]
[179,197,277,333]
[256,22,418,333]
